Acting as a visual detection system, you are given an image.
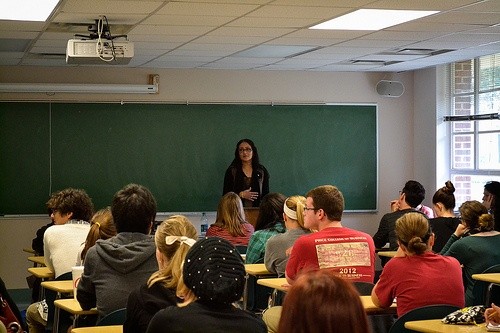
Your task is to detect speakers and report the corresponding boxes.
[376,80,405,98]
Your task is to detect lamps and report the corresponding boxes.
[0,75,161,95]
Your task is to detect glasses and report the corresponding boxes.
[399,190,404,195]
[487,181,498,196]
[304,206,319,212]
[238,148,252,153]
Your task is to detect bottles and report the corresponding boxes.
[199,212,209,237]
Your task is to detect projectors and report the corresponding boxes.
[66,39,135,65]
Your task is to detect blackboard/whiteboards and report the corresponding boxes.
[0,100,382,217]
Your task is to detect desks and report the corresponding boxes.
[24,246,500,333]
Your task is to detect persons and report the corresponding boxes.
[25,187,96,333]
[370,212,465,333]
[74,206,116,267]
[425,181,470,253]
[276,271,370,333]
[144,236,268,333]
[241,192,288,313]
[264,194,319,307]
[205,192,255,247]
[262,185,376,333]
[371,180,428,287]
[222,138,270,206]
[122,214,199,333]
[439,200,500,307]
[67,184,159,333]
[480,181,500,232]
[483,304,500,325]
[414,204,434,219]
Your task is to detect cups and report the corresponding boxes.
[72,267,84,300]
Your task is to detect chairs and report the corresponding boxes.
[348,281,395,333]
[472,264,500,306]
[46,270,73,333]
[247,257,278,310]
[388,303,461,333]
[96,306,127,326]
